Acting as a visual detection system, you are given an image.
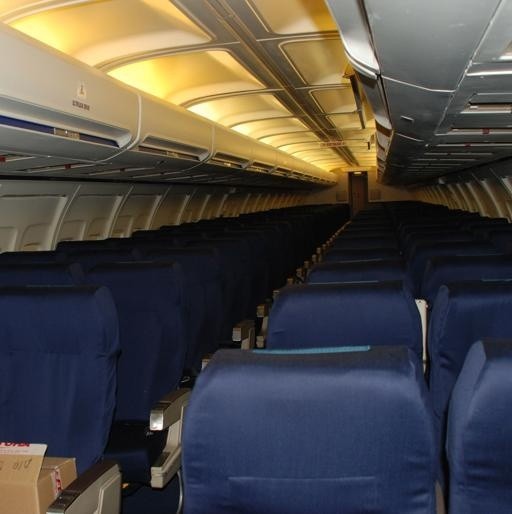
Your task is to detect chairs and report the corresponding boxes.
[2,202,510,513]
